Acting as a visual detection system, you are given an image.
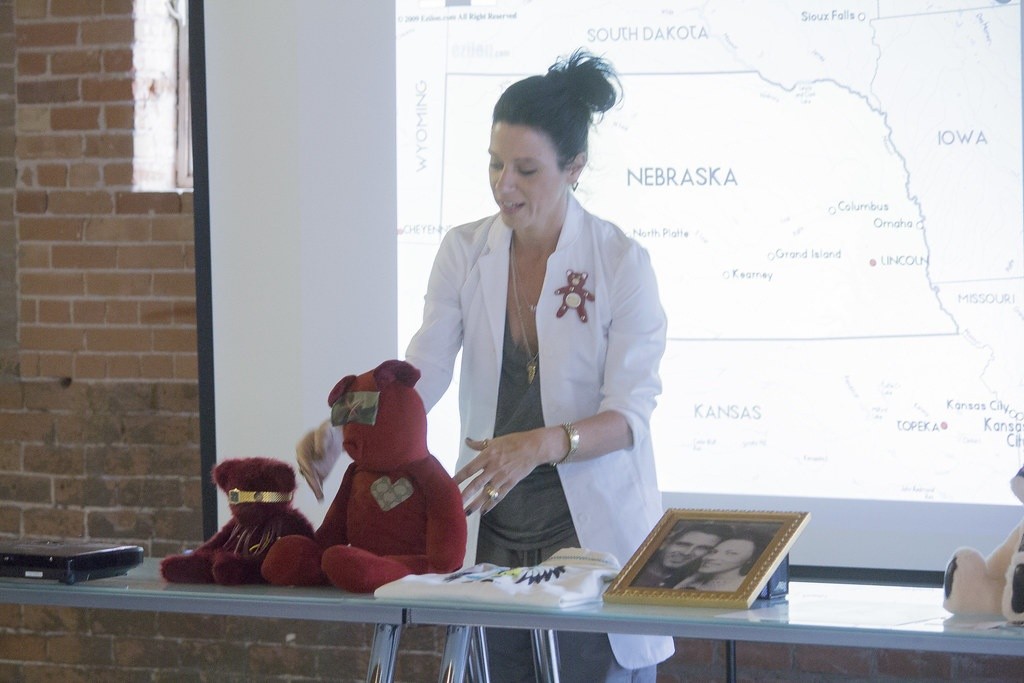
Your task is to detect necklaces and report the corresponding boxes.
[510,240,539,384]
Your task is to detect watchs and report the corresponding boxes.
[558,422,579,464]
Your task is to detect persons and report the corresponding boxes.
[297,45,675,683]
[629,523,773,592]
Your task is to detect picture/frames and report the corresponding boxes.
[603,508,811,609]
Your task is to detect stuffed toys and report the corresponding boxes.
[943,465,1024,623]
[261,360,468,592]
[161,457,317,586]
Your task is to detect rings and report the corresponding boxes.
[300,468,303,475]
[485,486,498,499]
[484,439,488,448]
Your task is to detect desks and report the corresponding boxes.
[0,557,491,683]
[407,581,1024,683]
